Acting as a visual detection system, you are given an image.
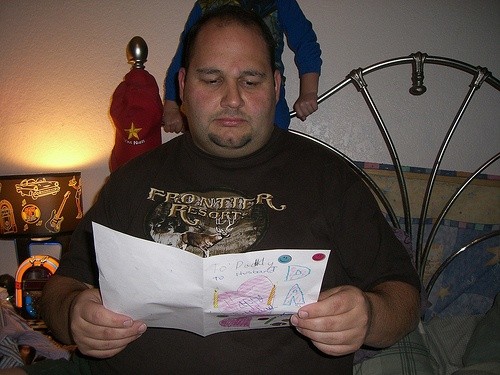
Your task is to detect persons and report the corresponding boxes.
[161,0,322,131]
[39,1,425,373]
